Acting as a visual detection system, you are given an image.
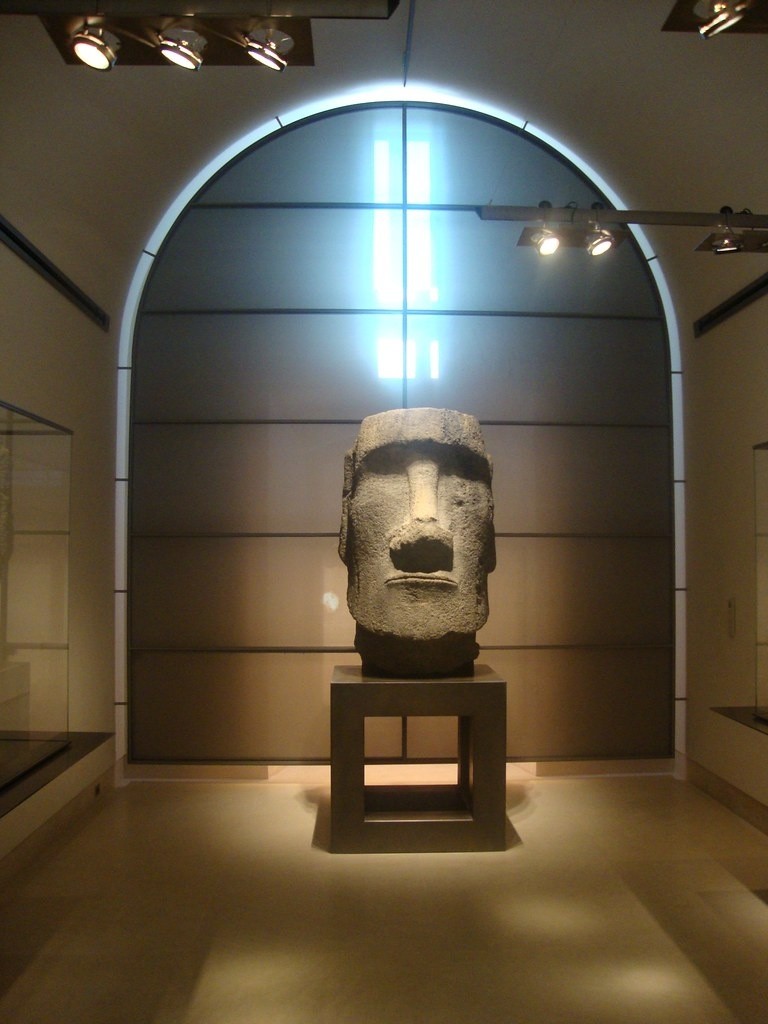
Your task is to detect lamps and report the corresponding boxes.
[517,204,762,254]
[71,17,295,72]
[694,0,748,40]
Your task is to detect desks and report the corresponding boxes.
[323,663,513,853]
